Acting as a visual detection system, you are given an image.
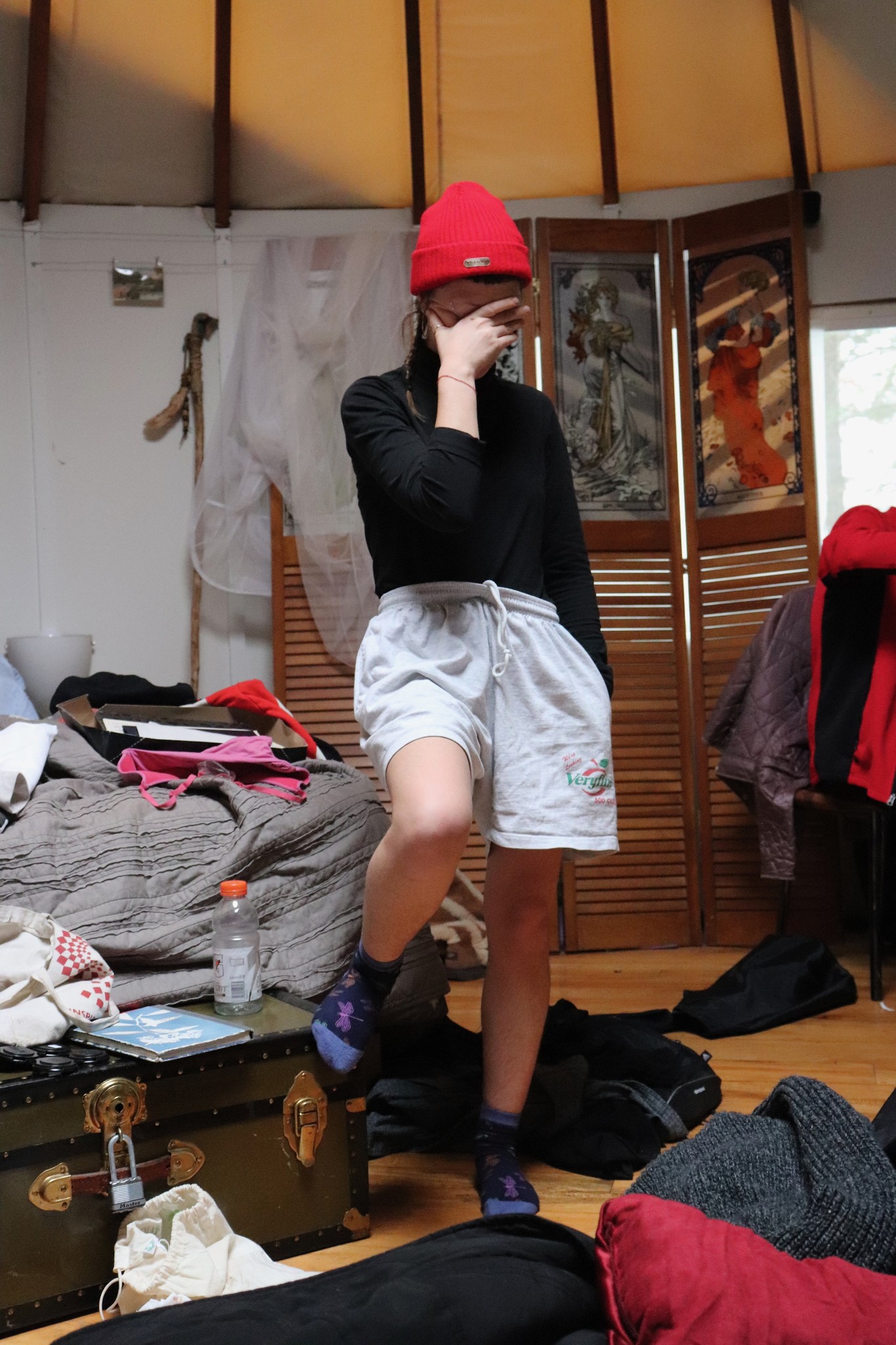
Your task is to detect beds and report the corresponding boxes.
[0,627,450,1053]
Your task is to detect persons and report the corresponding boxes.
[310,183,619,1218]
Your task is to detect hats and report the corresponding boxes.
[410,181,532,295]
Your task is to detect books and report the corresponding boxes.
[67,1004,255,1063]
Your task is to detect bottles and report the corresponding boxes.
[211,881,265,1018]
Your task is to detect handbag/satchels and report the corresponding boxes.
[98,1184,324,1322]
[0,906,121,1048]
[116,736,309,808]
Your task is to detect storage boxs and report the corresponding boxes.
[0,986,376,1341]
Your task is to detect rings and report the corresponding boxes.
[434,324,444,328]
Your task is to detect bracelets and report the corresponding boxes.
[437,375,478,393]
